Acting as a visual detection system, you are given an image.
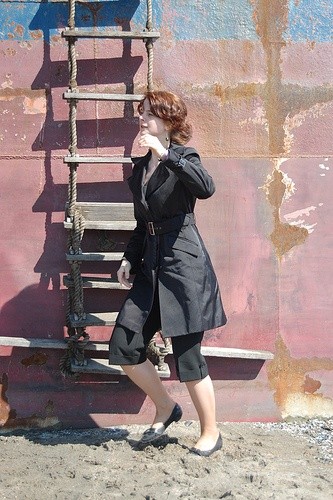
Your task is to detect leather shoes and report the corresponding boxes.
[187,432,223,458]
[140,402,183,443]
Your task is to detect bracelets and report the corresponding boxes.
[158,148,166,160]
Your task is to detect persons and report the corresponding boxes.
[108,91,228,456]
